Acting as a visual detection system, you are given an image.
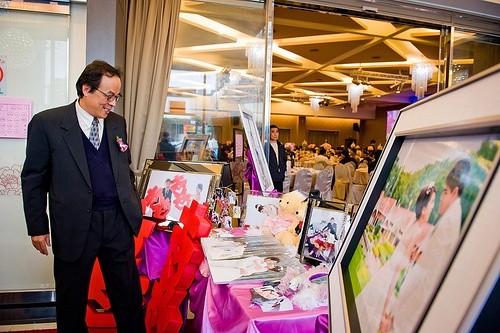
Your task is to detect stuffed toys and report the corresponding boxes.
[262,189,308,247]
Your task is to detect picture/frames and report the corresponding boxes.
[179,135,210,161]
[297,206,353,266]
[137,159,224,233]
[328,64,500,333]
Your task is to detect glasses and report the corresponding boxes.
[94,87,122,102]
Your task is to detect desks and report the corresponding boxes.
[180,227,329,333]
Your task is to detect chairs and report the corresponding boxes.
[283,164,369,214]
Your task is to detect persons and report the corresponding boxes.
[212,141,234,162]
[154,179,172,216]
[322,217,338,240]
[353,159,471,333]
[264,125,287,194]
[20,60,148,333]
[159,132,175,160]
[189,183,203,205]
[286,140,383,174]
[211,254,284,283]
[210,241,247,259]
[255,204,278,216]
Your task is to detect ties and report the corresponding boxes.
[89,116,101,149]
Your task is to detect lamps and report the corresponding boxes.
[346,84,368,113]
[309,97,324,119]
[245,43,278,77]
[229,72,241,90]
[409,63,433,100]
